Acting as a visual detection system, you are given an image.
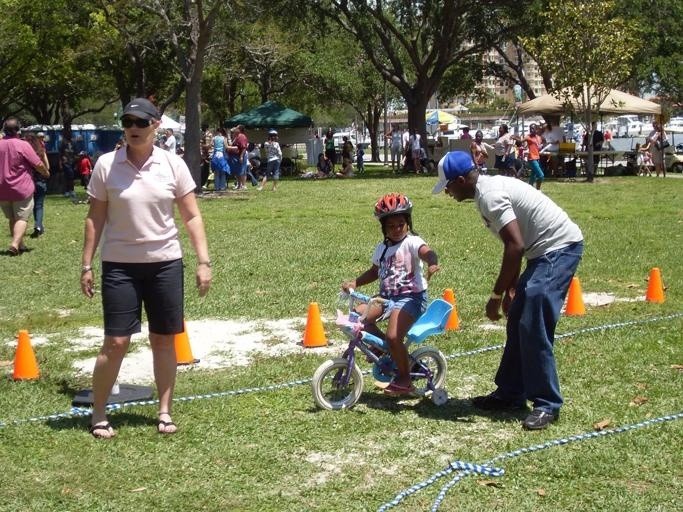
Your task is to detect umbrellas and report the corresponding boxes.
[425,111,456,126]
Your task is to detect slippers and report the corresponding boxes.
[157,421,177,434]
[89,422,115,439]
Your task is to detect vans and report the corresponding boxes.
[322,132,360,147]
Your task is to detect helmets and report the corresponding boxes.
[372,192,413,220]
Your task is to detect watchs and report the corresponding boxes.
[199,260,212,266]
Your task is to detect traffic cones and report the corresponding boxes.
[13,329,41,380]
[564,275,586,317]
[174,319,200,365]
[302,302,333,348]
[443,288,462,331]
[646,268,665,303]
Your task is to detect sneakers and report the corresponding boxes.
[384,378,416,395]
[19,246,33,252]
[473,394,526,410]
[523,409,559,430]
[8,247,18,256]
[30,227,41,238]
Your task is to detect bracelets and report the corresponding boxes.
[82,265,93,272]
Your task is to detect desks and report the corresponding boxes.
[540,150,638,178]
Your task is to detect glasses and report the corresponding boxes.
[122,118,153,128]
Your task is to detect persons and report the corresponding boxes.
[431,150,583,429]
[384,123,428,175]
[80,98,213,439]
[603,130,630,142]
[492,124,566,192]
[636,121,668,178]
[59,150,93,198]
[583,122,605,176]
[0,117,50,255]
[343,193,440,394]
[468,130,496,175]
[115,127,177,156]
[460,128,472,139]
[316,128,365,178]
[200,120,282,191]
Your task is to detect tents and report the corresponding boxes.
[223,100,316,174]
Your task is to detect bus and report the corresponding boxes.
[16,124,159,181]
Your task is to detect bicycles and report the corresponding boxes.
[311,288,454,410]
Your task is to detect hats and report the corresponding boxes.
[119,97,161,121]
[431,151,477,194]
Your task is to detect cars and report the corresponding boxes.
[601,114,683,138]
